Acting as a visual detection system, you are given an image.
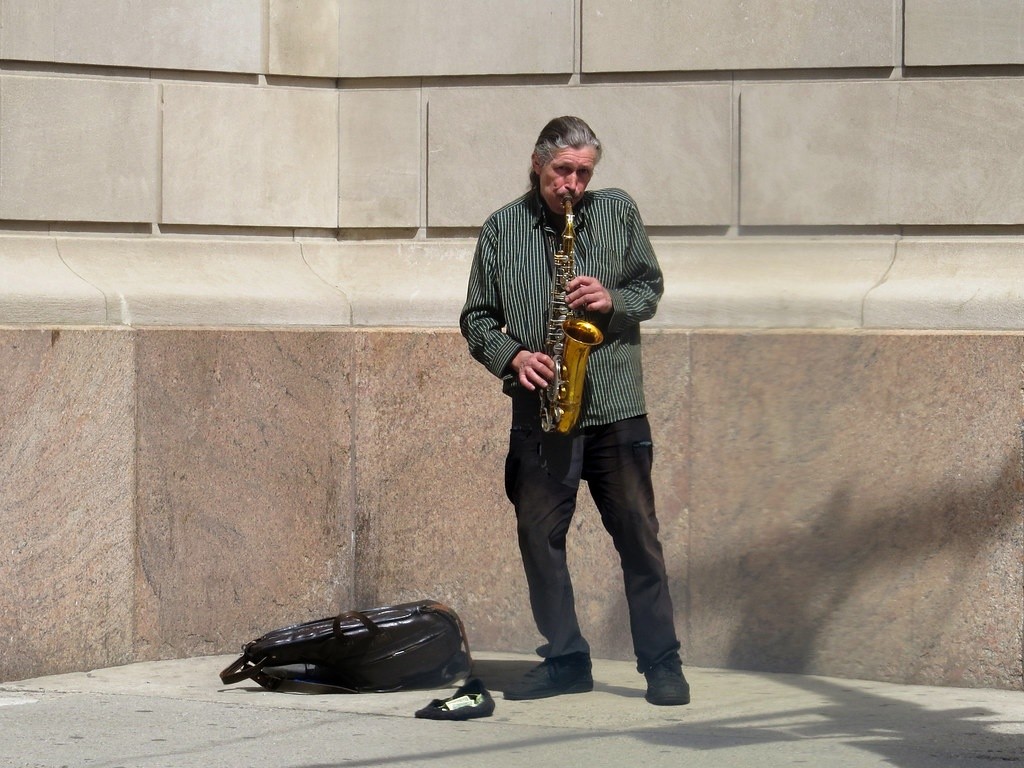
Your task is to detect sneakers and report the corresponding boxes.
[502,653,594,700]
[644,664,690,706]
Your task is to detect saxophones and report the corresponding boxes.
[538,193,603,434]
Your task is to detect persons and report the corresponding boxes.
[459,117,689,705]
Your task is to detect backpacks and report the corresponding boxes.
[218,599,474,695]
